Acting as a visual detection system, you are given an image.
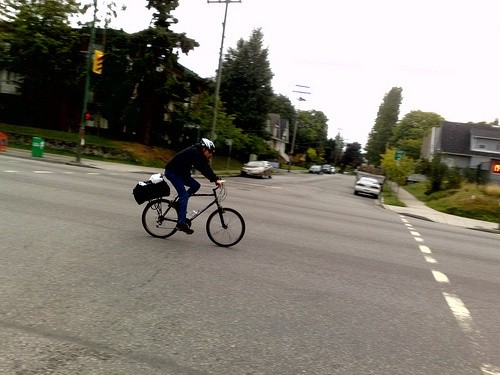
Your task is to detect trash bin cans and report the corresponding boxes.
[32,137,45,158]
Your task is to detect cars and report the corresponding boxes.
[309,165,322,173]
[322,164,331,174]
[242,161,273,180]
[354,178,381,197]
[331,167,339,173]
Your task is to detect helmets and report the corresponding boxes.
[202,137,217,156]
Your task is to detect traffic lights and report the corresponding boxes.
[92,49,106,77]
[84,113,95,121]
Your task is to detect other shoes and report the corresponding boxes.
[176,223,194,235]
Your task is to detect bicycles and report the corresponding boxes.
[141,178,249,246]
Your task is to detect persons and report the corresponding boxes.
[164,138,224,235]
